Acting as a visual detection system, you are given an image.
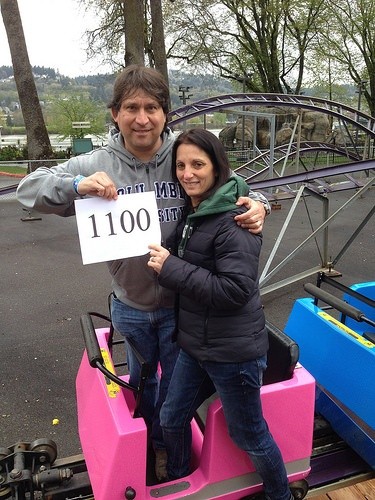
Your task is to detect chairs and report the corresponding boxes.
[196,320,299,438]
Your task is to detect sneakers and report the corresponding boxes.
[155,444,168,480]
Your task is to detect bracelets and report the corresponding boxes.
[262,202,270,217]
[74,174,85,195]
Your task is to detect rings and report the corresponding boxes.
[258,221,261,226]
[150,256,154,261]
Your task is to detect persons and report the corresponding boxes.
[15,64,272,485]
[147,129,295,500]
